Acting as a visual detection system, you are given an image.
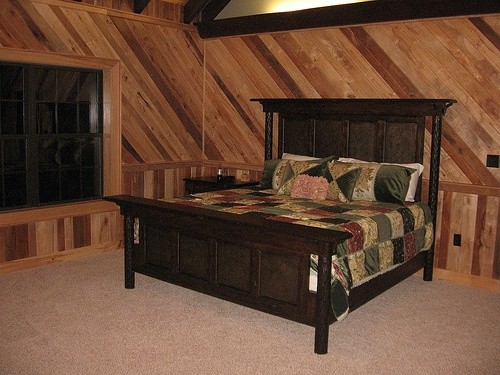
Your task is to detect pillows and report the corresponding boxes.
[278,156,359,205]
[332,158,415,206]
[341,155,427,202]
[293,173,328,202]
[262,152,335,190]
[282,151,322,160]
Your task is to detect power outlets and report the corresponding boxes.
[453,234,461,246]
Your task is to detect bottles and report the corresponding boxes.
[217,168,222,181]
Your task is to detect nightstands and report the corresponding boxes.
[181,173,260,195]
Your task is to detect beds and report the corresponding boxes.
[97,98,459,355]
